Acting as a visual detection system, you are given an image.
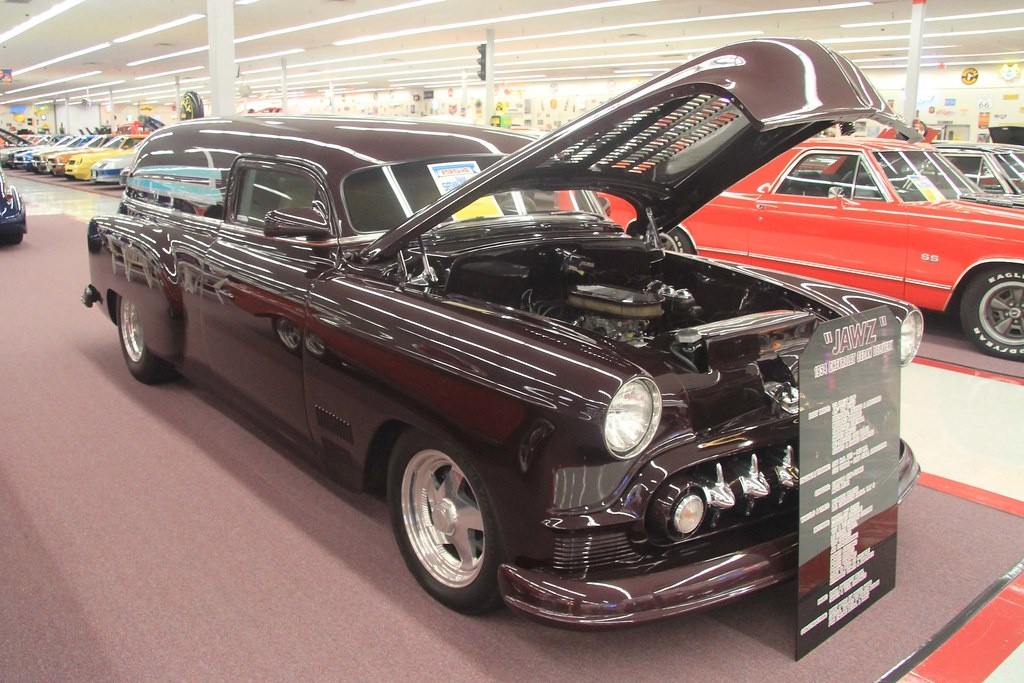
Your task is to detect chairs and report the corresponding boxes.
[788,172,841,197]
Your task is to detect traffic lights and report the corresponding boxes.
[476,44,486,79]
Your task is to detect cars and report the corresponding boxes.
[81,37,914,626]
[554,133,1024,363]
[0,128,148,185]
[772,141,1024,211]
[0,170,29,250]
